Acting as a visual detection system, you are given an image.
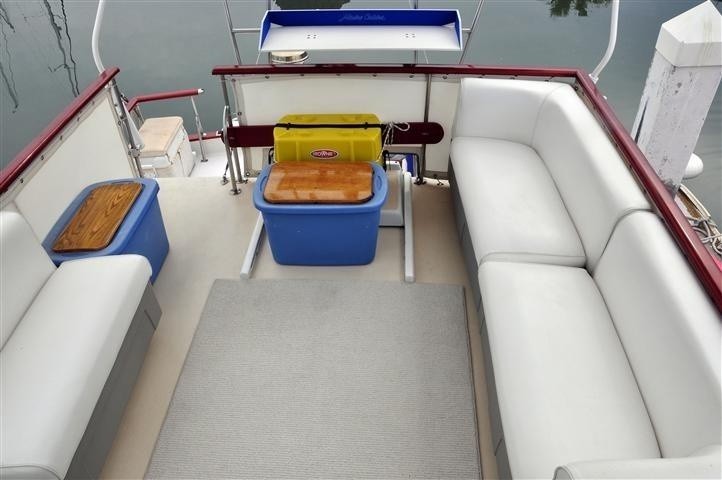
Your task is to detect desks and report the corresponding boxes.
[133,116,196,179]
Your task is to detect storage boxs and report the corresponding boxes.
[39,177,170,287]
[252,162,389,266]
[273,113,383,169]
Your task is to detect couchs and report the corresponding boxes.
[444,78,655,290]
[2,210,163,480]
[471,209,721,479]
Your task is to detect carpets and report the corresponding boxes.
[140,278,484,478]
